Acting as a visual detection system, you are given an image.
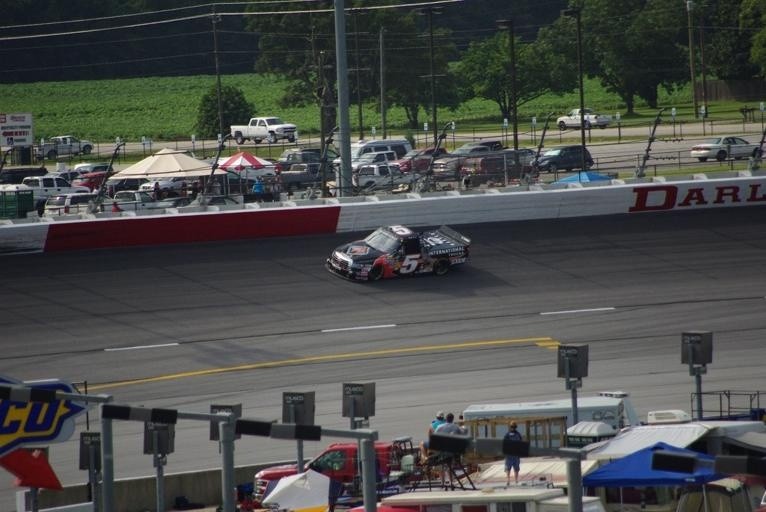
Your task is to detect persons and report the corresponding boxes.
[92,169,282,212]
[417,411,522,486]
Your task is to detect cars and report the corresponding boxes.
[691,136,764,162]
[325,224,471,283]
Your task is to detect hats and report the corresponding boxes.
[510,420,517,428]
[436,411,445,419]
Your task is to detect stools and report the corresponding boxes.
[393,435,414,458]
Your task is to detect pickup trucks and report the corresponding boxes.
[253,436,420,505]
[33,134,94,160]
[230,117,297,144]
[556,108,612,129]
